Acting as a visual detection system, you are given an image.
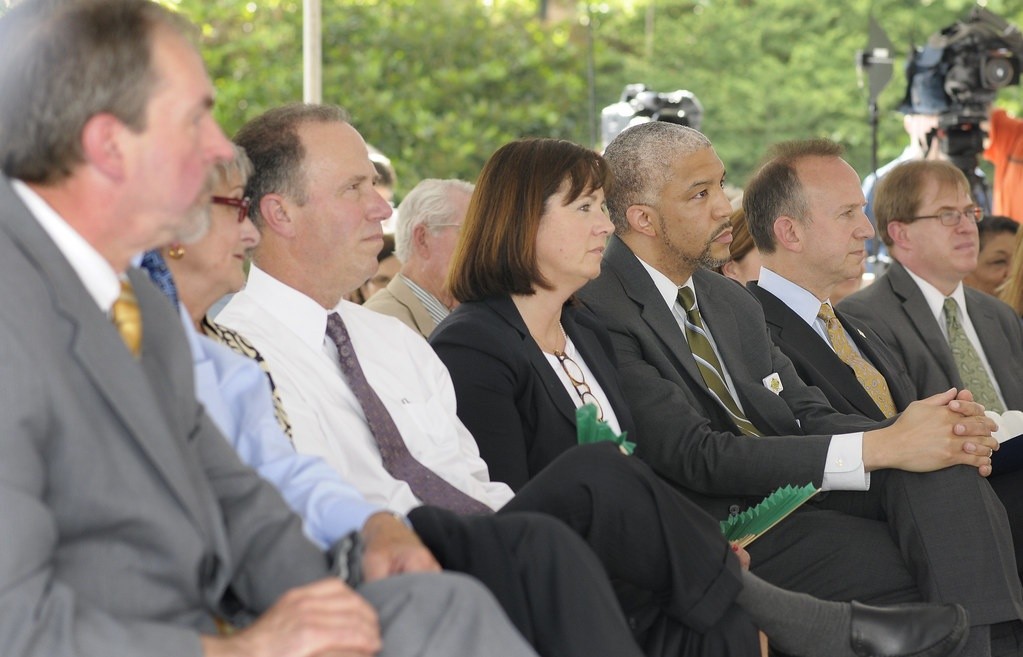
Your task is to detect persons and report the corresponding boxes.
[562,120,1023,656]
[0,0,1023,657]
[206,103,970,657]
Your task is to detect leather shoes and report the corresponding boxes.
[766,599,968,657]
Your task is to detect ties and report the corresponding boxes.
[942,298,1005,417]
[112,281,145,362]
[818,302,898,418]
[675,285,766,438]
[325,310,498,519]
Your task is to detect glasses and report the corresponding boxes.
[554,349,604,423]
[906,204,984,227]
[208,196,250,224]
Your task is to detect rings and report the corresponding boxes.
[986,448,992,457]
